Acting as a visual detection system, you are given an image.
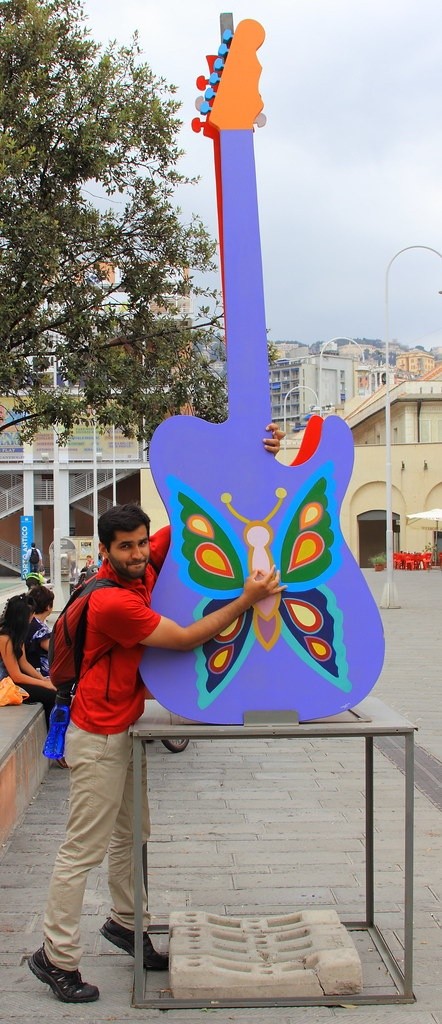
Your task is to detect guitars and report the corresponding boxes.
[132,19,385,727]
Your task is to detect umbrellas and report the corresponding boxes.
[406,508,442,567]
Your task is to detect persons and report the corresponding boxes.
[25,542,43,573]
[69,560,80,595]
[28,422,286,999]
[26,567,46,590]
[23,586,55,677]
[0,593,68,768]
[86,555,94,570]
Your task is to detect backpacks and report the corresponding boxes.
[48,574,124,689]
[29,548,39,564]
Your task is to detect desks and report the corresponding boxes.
[129,696,420,1009]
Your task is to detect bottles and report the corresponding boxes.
[36,668,41,674]
[43,694,72,759]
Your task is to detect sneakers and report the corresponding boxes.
[28,942,99,1002]
[99,917,169,969]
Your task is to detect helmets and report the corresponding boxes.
[26,572,45,590]
